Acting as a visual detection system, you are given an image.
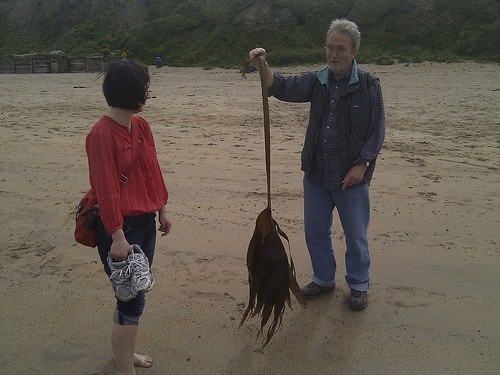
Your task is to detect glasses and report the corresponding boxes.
[324,45,355,53]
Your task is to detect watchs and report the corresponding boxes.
[365,161,370,167]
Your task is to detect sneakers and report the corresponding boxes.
[107,251,137,303]
[125,244,155,296]
[350,288,367,310]
[301,281,336,300]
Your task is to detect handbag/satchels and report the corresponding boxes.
[75,188,99,248]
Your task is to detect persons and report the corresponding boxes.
[250,20,385,311]
[86,59,172,375]
